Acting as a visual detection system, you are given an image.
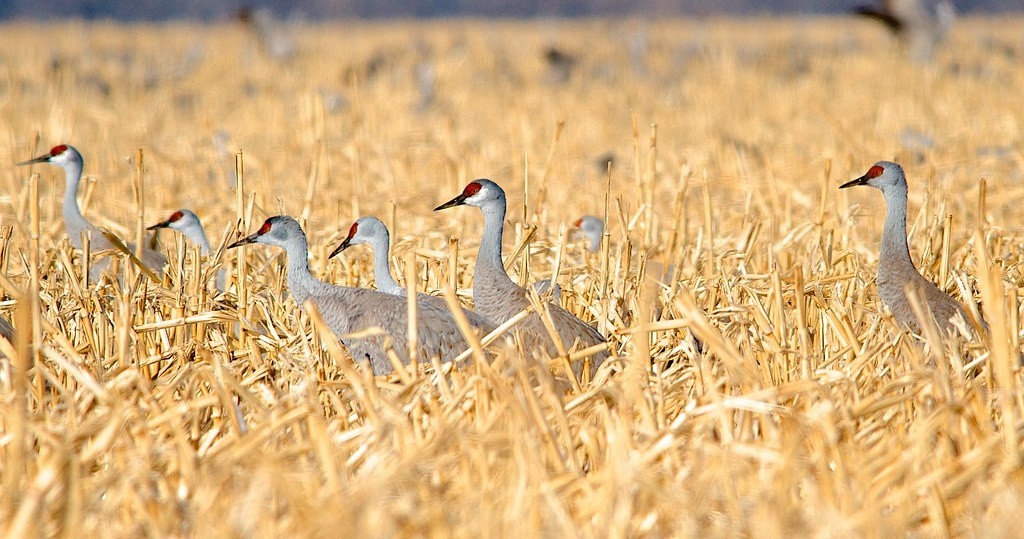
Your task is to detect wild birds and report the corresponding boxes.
[146,209,268,360]
[327,217,513,340]
[568,213,678,285]
[16,143,169,289]
[432,178,611,392]
[838,160,988,369]
[226,216,514,377]
